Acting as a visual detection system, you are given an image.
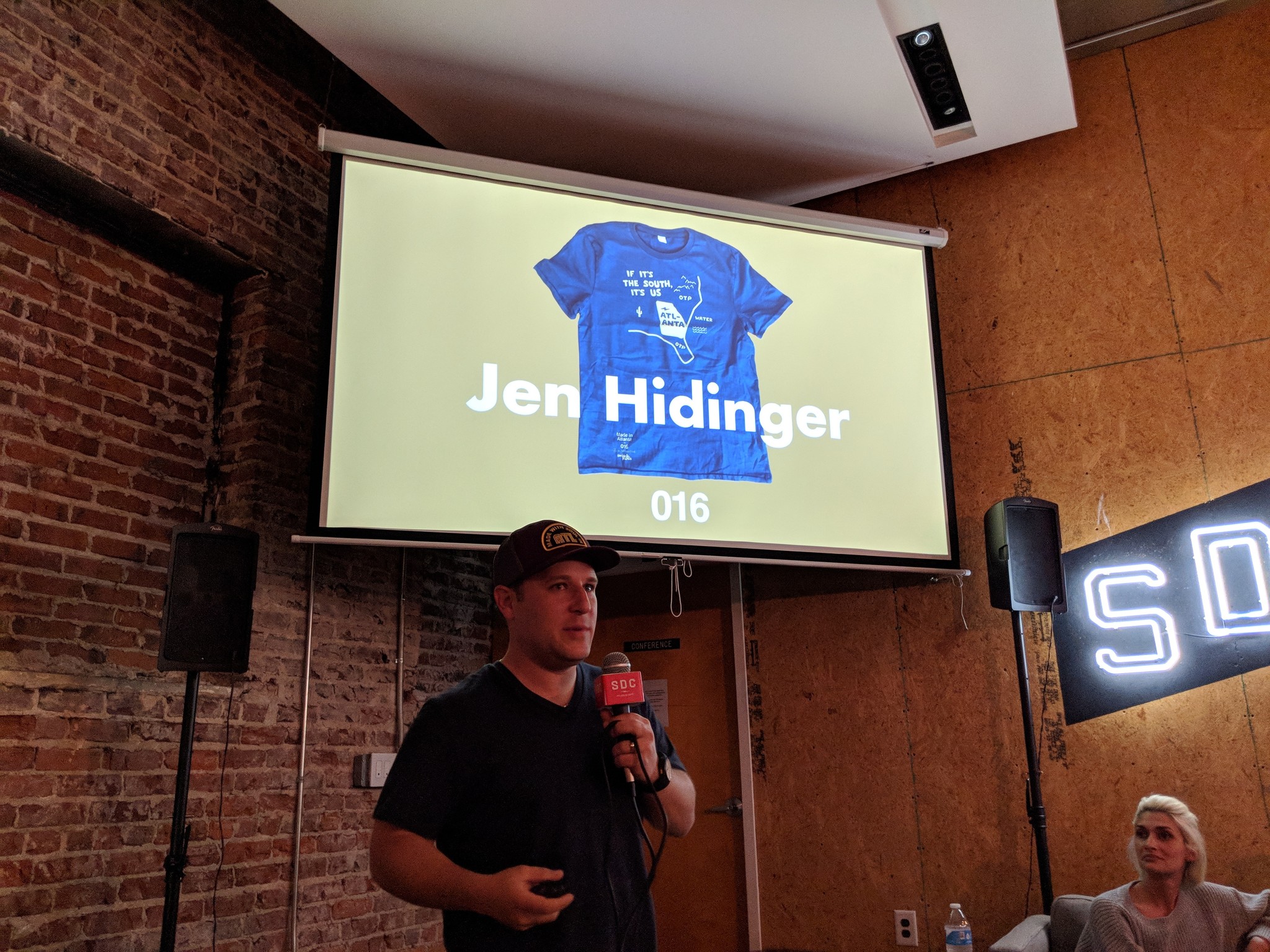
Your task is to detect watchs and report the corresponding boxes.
[638,751,673,793]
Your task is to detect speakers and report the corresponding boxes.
[155,523,260,671]
[985,498,1071,615]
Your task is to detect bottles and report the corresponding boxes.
[945,903,973,952]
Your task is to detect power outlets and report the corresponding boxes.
[894,910,919,947]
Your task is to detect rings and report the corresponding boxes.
[631,742,635,753]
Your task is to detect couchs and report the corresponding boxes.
[988,894,1096,952]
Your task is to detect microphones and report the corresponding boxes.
[596,653,646,785]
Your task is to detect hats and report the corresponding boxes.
[492,520,621,586]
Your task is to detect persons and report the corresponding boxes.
[369,519,696,952]
[1075,796,1270,952]
